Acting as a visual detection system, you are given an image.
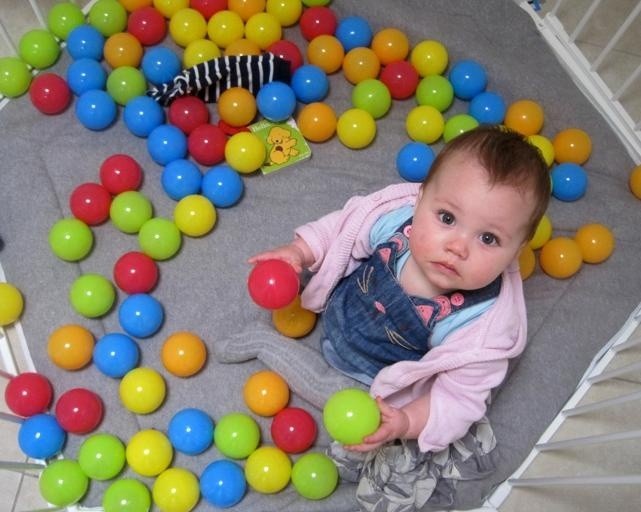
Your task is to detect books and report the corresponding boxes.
[246,115,312,176]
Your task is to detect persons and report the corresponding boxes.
[213,126,551,511]
